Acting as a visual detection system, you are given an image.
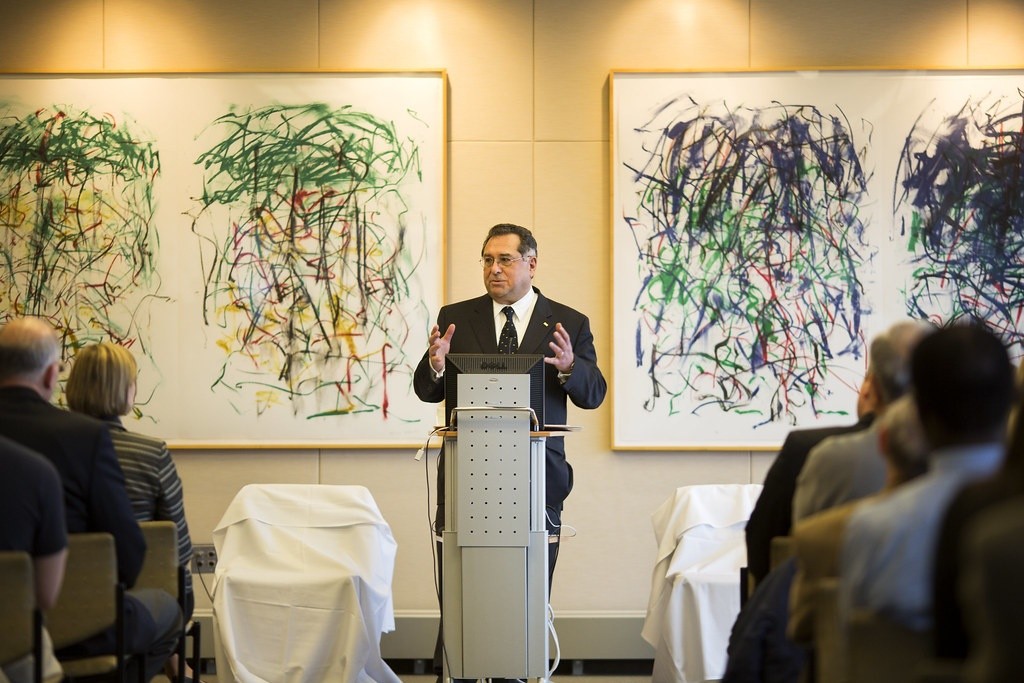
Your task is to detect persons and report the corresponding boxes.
[62,342,198,682]
[718,315,1024,683]
[0,316,186,683]
[0,435,74,682]
[412,222,606,683]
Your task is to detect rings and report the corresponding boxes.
[556,352,565,359]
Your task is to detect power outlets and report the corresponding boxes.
[191,544,219,573]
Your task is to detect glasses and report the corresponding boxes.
[479,257,532,265]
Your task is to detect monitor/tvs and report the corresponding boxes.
[444,352,545,431]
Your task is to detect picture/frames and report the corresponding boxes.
[0,69,452,446]
[607,67,1023,452]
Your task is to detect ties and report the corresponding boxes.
[497,306,519,355]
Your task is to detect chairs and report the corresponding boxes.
[0,548,43,683]
[45,530,145,682]
[133,517,204,682]
